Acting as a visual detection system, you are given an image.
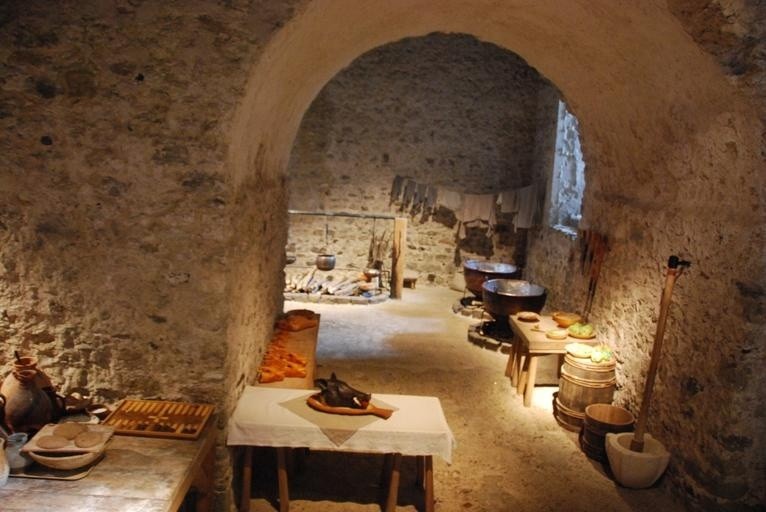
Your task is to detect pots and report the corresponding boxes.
[463,260,548,324]
[318,253,334,270]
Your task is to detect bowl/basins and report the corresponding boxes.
[554,312,582,327]
[28,436,113,471]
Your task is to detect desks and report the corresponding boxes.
[504,312,601,408]
[230,383,452,512]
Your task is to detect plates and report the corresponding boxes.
[568,328,596,339]
[84,402,109,418]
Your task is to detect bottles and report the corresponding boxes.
[5,432,34,469]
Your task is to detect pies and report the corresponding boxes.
[75,431,102,448]
[53,421,87,440]
[37,435,68,449]
